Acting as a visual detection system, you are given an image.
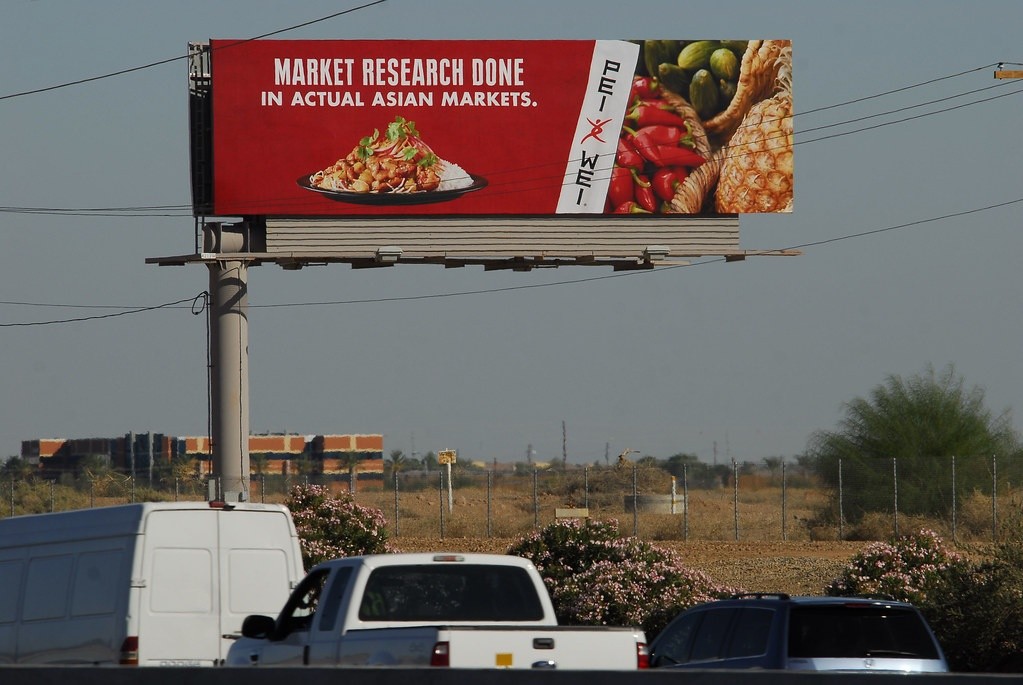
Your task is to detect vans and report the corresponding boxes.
[1,498,303,668]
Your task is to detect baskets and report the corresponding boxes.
[661,40,789,215]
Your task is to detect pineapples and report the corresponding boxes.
[714,58,793,213]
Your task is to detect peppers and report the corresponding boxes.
[603,76,706,214]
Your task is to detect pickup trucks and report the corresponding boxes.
[219,551,650,671]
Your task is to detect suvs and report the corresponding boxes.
[645,589,948,671]
